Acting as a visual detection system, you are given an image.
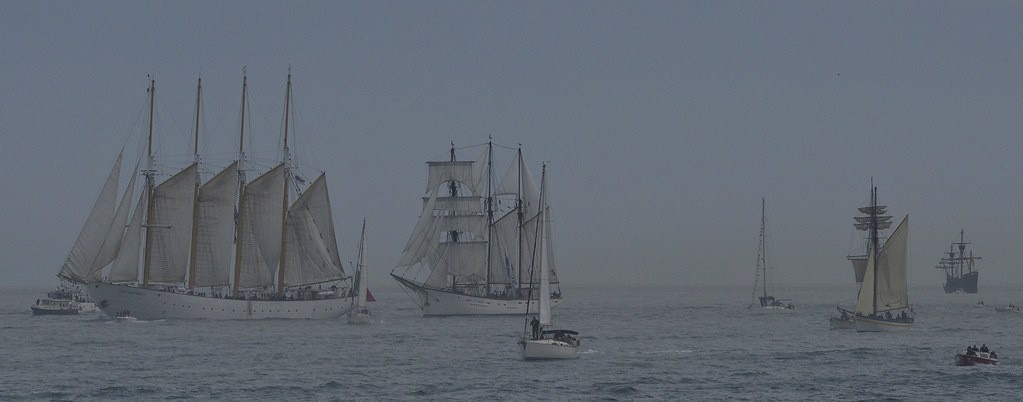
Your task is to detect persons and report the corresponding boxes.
[530,316,540,340]
[966,344,997,360]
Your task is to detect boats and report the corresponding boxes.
[31,273,101,316]
[952,343,1001,368]
[116,316,138,323]
[995,304,1020,314]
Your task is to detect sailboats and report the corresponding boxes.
[830,177,917,333]
[933,231,981,296]
[346,218,373,324]
[391,133,564,318]
[519,165,581,362]
[54,64,361,324]
[745,196,797,314]
